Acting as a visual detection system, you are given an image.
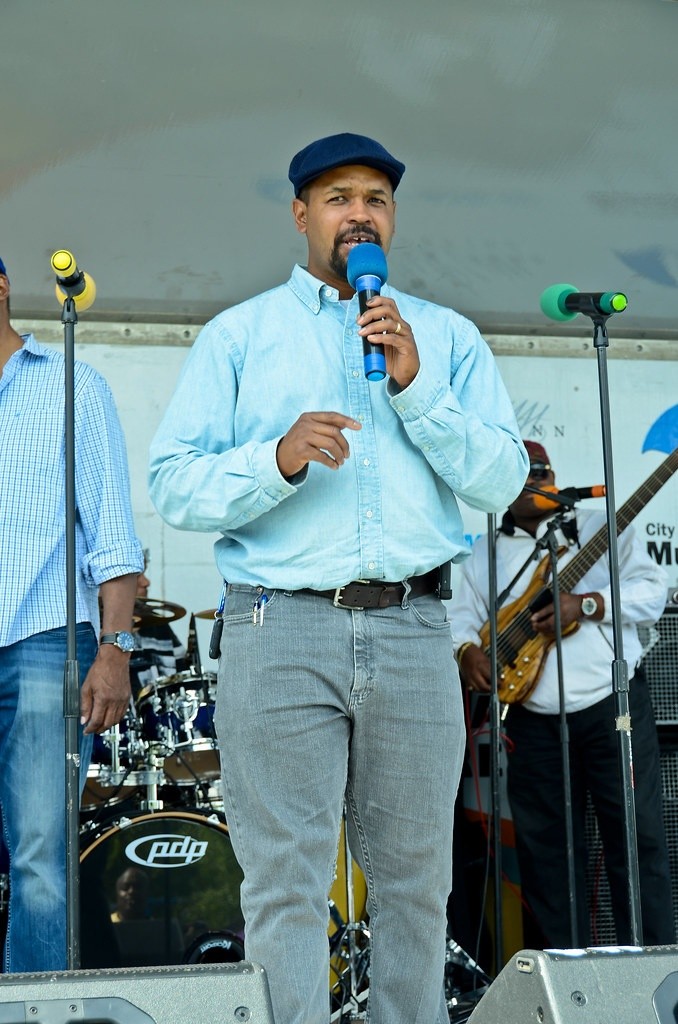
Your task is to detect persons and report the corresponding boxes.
[1,264,149,975]
[149,131,530,1024]
[448,439,667,950]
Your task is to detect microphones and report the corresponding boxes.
[187,614,196,653]
[540,283,628,323]
[533,485,607,509]
[51,249,95,313]
[347,242,389,382]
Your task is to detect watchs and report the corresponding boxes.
[579,593,597,618]
[99,630,137,654]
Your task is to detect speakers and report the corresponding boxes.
[463,606,678,1024]
[0,960,277,1024]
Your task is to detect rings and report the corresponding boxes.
[394,322,402,333]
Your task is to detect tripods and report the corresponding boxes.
[328,827,371,1013]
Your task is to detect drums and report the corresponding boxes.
[78,702,145,812]
[327,801,368,996]
[138,669,222,785]
[75,804,247,968]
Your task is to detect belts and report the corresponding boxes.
[293,561,440,611]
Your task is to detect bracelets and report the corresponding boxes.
[458,641,473,665]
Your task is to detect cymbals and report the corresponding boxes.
[98,595,188,629]
[193,610,223,620]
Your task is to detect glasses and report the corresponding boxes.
[529,463,548,481]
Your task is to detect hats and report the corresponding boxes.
[523,440,551,466]
[289,133,406,198]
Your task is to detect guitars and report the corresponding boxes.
[480,445,678,707]
